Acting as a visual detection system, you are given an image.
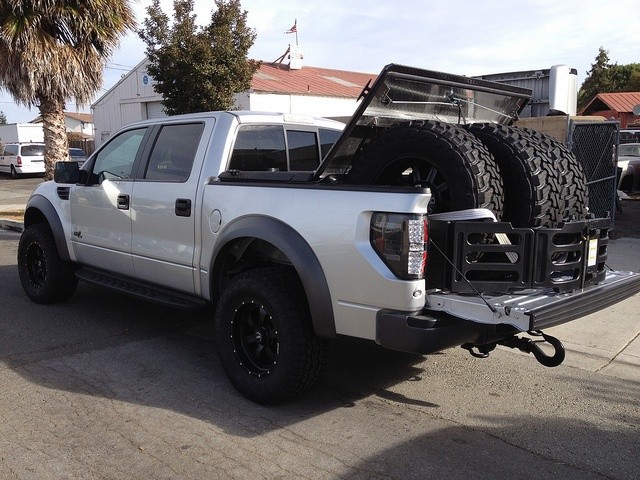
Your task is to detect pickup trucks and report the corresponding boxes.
[18,63,640,408]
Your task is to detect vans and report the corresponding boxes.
[0,142,46,179]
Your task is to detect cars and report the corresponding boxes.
[68,147,86,168]
